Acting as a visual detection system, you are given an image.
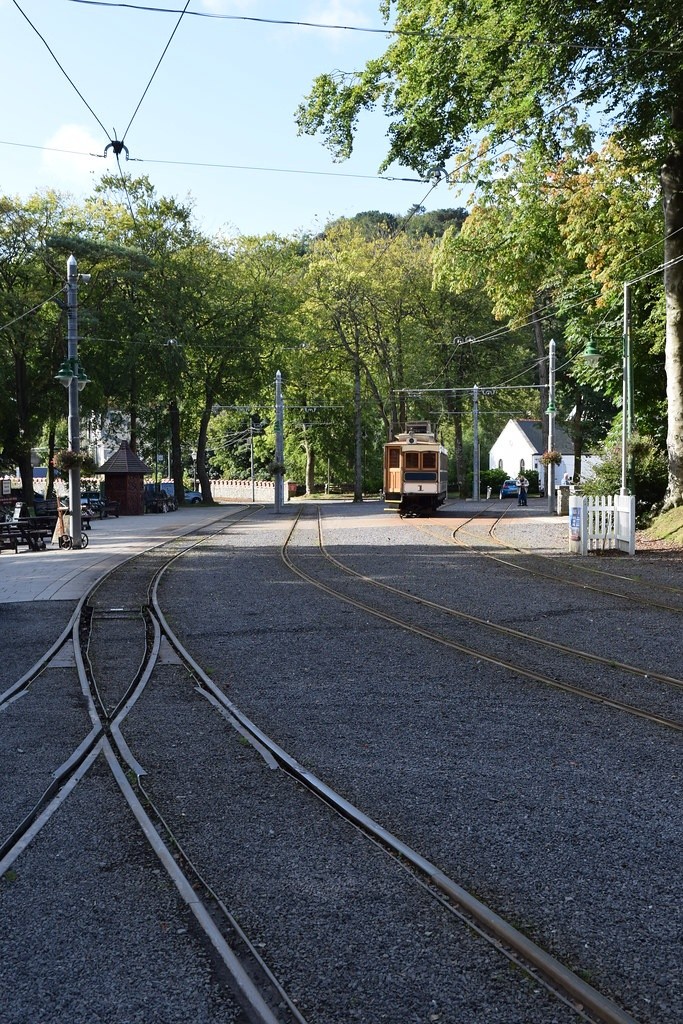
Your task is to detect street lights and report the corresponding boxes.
[544,402,561,513]
[273,419,285,513]
[579,331,629,496]
[54,354,91,548]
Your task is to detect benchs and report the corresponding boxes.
[0,487,180,552]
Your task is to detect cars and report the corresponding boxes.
[182,486,202,504]
[499,480,520,499]
[80,490,103,504]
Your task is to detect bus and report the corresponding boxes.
[381,420,449,518]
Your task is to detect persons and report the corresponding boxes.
[516,475,529,505]
